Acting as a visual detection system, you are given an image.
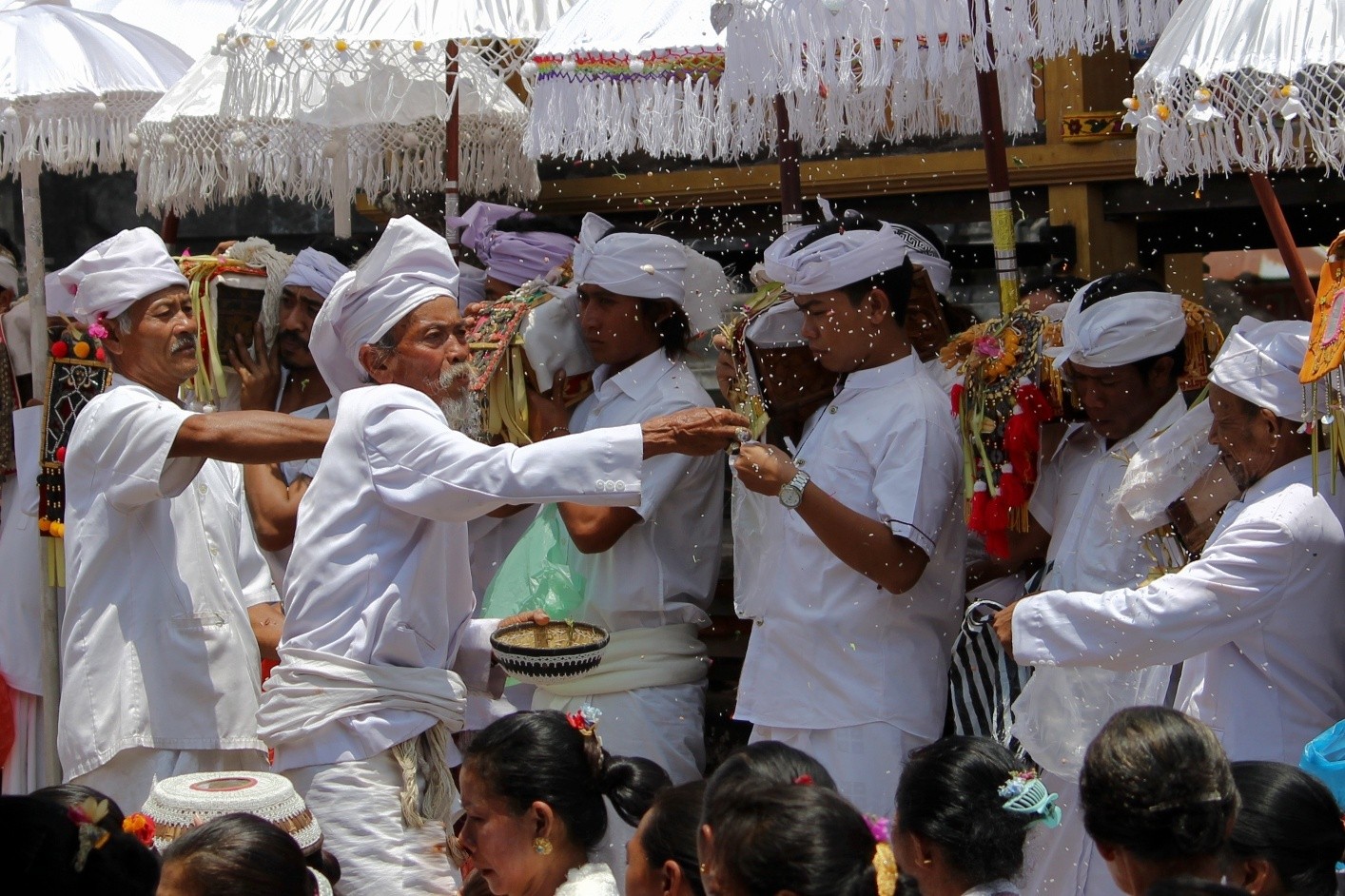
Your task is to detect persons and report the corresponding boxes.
[0,209,1344,896]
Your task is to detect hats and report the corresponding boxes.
[132,771,325,864]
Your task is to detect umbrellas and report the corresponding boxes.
[0,0,1345,209]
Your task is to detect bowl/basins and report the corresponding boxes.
[489,621,608,685]
